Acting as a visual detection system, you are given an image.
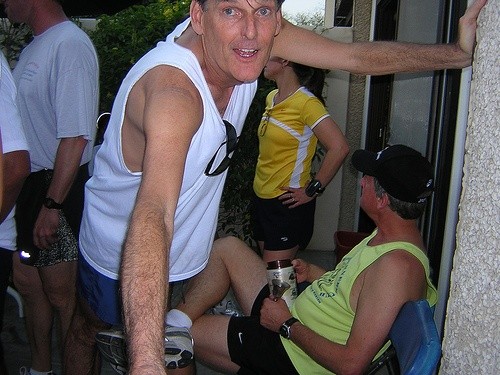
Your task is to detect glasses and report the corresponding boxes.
[260,110,272,136]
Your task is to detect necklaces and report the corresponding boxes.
[275,87,297,103]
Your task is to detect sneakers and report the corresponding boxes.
[95,321,194,369]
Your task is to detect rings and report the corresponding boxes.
[291,193,292,196]
[293,198,295,201]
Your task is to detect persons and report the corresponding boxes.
[0,0,104,375]
[163,145,439,375]
[250,55,351,265]
[0,50,31,375]
[63,0,488,375]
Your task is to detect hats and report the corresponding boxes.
[350,143,437,200]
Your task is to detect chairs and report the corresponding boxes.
[363,299,443,375]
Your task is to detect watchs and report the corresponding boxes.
[42,197,62,209]
[306,180,324,198]
[280,317,298,339]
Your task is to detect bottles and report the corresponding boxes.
[266,258,297,308]
